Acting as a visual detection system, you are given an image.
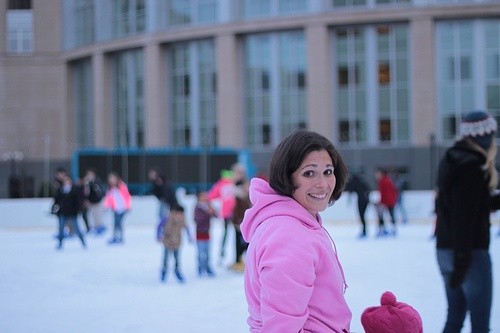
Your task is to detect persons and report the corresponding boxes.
[51,169,132,252]
[142,162,251,283]
[432,111,500,333]
[361,291,424,333]
[240,131,352,333]
[346,164,414,240]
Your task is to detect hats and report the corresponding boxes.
[460,111,497,151]
[361,291,423,333]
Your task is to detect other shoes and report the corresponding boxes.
[160,261,245,282]
[359,227,398,238]
[55,224,127,252]
